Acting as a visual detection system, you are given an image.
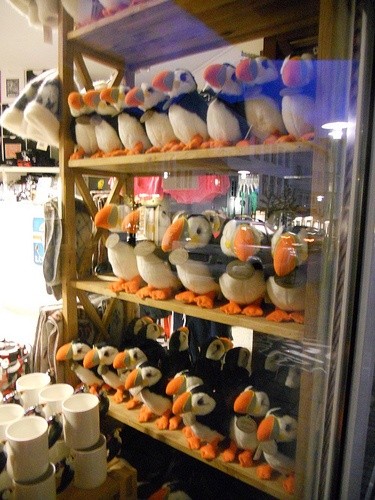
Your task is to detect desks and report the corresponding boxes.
[52,454,139,500]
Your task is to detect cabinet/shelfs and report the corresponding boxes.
[56,0,358,500]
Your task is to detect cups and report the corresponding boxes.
[0,372,107,500]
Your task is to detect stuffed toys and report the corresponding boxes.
[67,50,320,157]
[93,203,308,326]
[122,317,300,411]
[55,339,299,496]
